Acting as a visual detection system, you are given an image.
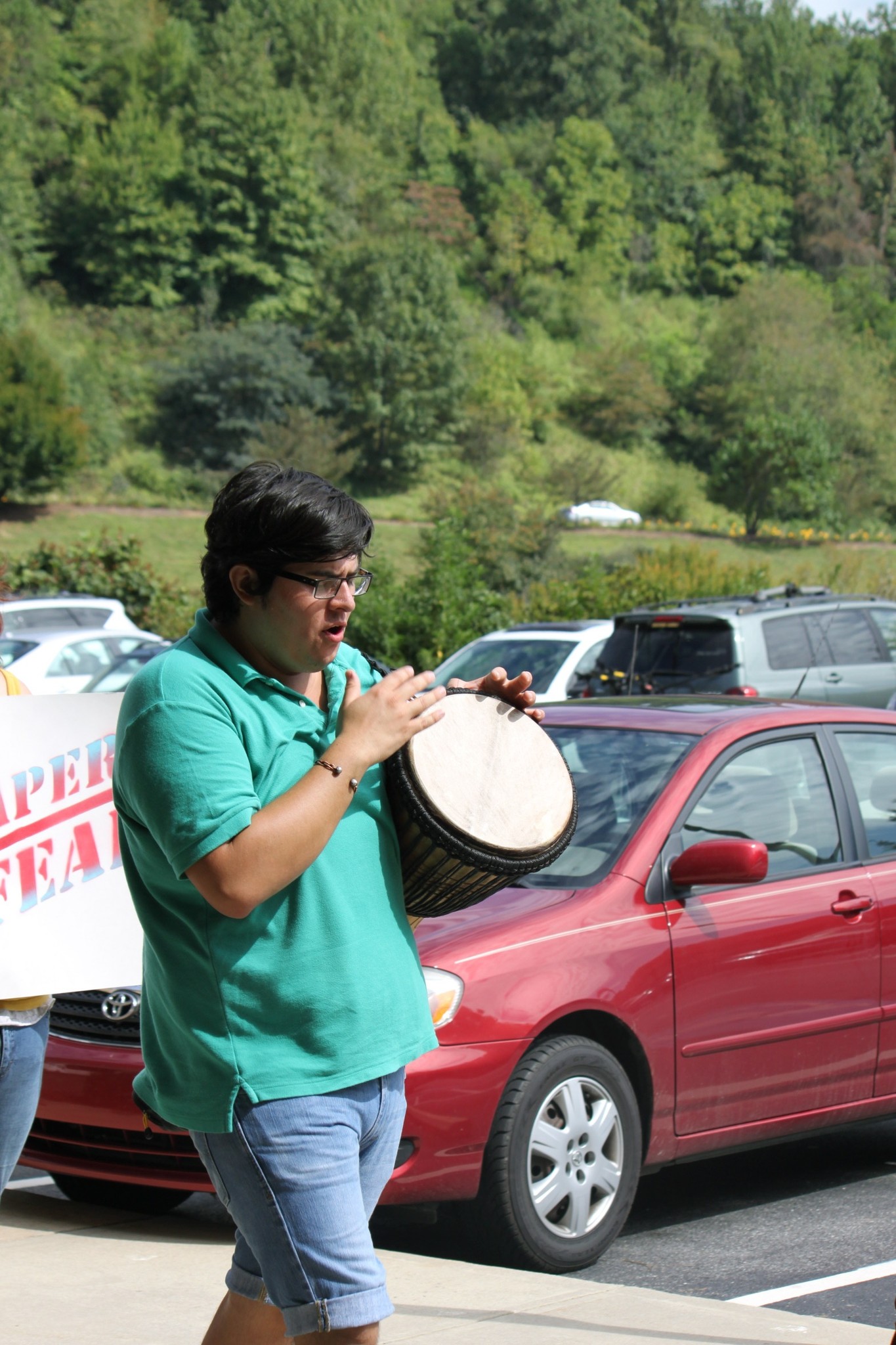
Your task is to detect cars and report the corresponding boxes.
[15,685,896,1278]
[415,618,614,706]
[561,500,642,530]
[0,596,170,698]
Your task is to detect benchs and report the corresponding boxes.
[774,761,896,862]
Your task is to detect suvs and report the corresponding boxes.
[570,583,895,774]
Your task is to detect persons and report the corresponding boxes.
[113,457,546,1345]
[0,658,56,1199]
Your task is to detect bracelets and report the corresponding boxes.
[314,760,358,793]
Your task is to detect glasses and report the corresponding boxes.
[277,568,373,600]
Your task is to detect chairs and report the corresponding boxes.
[569,772,617,848]
[707,766,813,877]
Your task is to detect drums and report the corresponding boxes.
[381,687,581,932]
